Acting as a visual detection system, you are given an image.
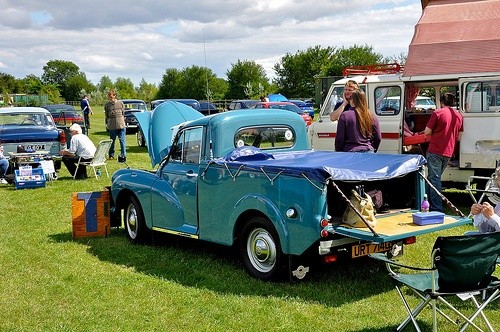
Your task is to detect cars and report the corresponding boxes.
[104,98,149,135]
[380,93,436,116]
[149,94,314,142]
[32,104,85,135]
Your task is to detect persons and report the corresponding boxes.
[330,80,360,121]
[424,92,464,214]
[105,90,127,160]
[335,90,381,153]
[81,95,95,128]
[471,167,500,235]
[0,140,9,184]
[59,123,98,179]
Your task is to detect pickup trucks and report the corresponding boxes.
[107,100,431,283]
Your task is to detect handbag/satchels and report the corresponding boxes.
[342,189,376,228]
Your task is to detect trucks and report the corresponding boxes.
[306,60,500,210]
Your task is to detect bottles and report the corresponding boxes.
[0,146,3,158]
[96,167,101,175]
[421,194,429,213]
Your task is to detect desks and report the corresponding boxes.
[8,156,64,180]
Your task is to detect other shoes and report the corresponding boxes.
[0,178,7,184]
[75,174,87,179]
[110,156,113,160]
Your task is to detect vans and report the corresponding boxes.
[0,106,67,173]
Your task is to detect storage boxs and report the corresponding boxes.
[412,210,445,225]
[14,169,47,190]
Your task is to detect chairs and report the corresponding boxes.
[73,139,113,181]
[466,173,500,219]
[367,231,500,332]
